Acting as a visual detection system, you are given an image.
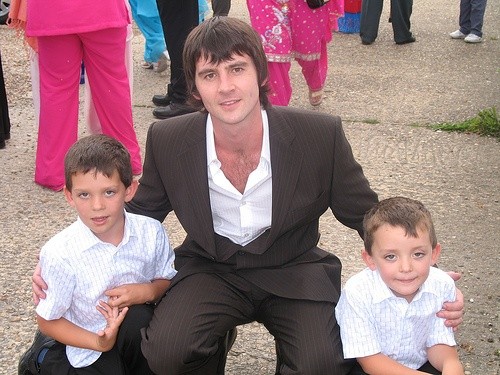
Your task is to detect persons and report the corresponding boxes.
[0,0,415,148]
[18,133,178,375]
[26,0,142,192]
[335,196,464,375]
[448,0,486,43]
[31,16,464,375]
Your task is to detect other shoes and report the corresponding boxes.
[396,38,415,43]
[464,34,482,42]
[142,61,160,71]
[450,30,467,38]
[309,90,322,105]
[361,41,369,45]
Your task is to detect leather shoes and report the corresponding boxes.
[154,103,195,119]
[152,95,174,106]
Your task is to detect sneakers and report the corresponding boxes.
[19,329,56,375]
